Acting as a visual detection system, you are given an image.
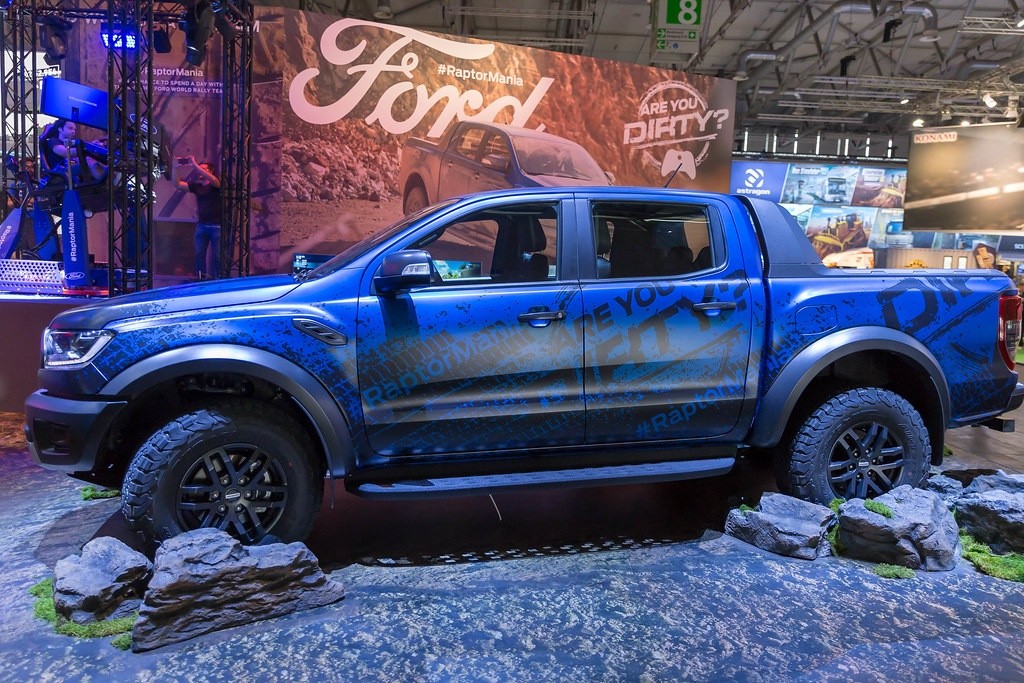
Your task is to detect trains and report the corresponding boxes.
[804,177,847,203]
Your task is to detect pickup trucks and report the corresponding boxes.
[21,185,1024,552]
[396,119,617,261]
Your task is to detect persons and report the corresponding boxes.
[45,118,123,188]
[7,157,49,259]
[170,155,223,280]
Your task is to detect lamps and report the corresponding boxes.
[1009,0,1024,28]
[182,0,215,67]
[981,93,996,108]
[888,146,898,158]
[34,14,73,64]
[735,139,744,151]
[840,55,856,76]
[374,0,393,18]
[883,20,902,43]
[145,15,171,54]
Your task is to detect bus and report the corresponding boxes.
[884,218,914,248]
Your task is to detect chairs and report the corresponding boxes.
[595,217,611,279]
[513,217,548,282]
[666,246,692,267]
[690,245,711,273]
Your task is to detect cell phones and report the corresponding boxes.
[178,158,189,164]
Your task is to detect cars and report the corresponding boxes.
[957,233,987,250]
